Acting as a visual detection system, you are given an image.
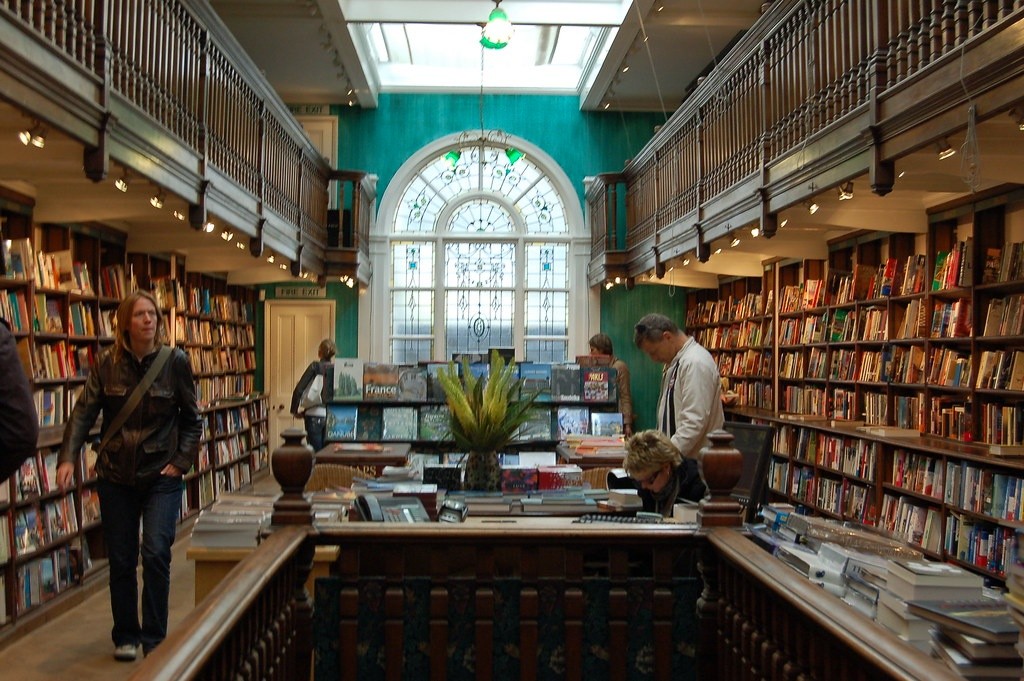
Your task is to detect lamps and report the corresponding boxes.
[440,55,526,169]
[602,179,855,290]
[305,0,358,107]
[1011,99,1024,132]
[20,118,48,148]
[115,170,356,286]
[480,0,514,52]
[933,135,956,163]
[599,0,664,110]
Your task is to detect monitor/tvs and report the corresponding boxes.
[722,421,776,508]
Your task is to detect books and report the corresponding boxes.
[683,236,1024,575]
[747,499,1024,681]
[325,358,629,461]
[190,463,643,546]
[0,237,273,623]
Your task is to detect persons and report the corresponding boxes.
[589,335,635,438]
[0,318,40,485]
[290,339,336,453]
[55,290,204,662]
[632,313,724,503]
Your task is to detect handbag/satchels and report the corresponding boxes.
[300,363,323,408]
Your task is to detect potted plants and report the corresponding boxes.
[425,351,543,490]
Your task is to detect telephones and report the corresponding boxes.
[354,495,431,523]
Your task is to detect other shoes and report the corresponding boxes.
[114,644,136,661]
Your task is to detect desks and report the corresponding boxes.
[557,443,627,468]
[186,546,342,633]
[315,442,412,479]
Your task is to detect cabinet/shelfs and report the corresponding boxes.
[0,184,273,648]
[680,181,1023,617]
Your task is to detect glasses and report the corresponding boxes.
[634,323,663,335]
[635,466,665,489]
